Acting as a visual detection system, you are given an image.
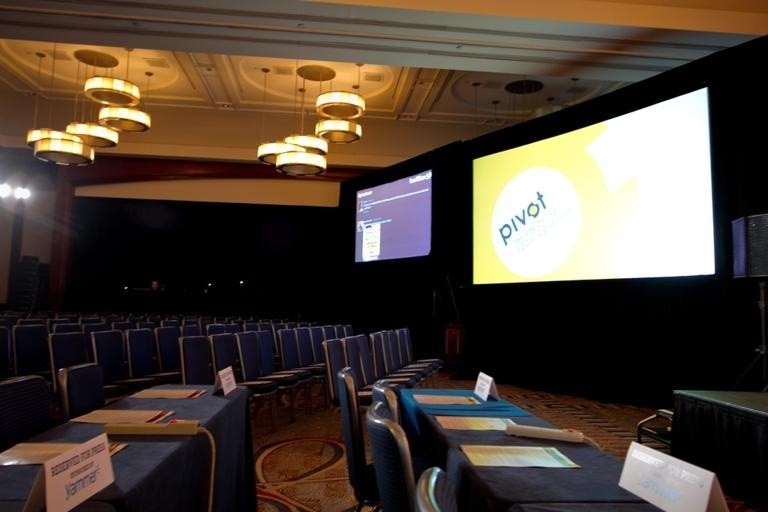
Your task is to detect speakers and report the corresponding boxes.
[732,212,768,278]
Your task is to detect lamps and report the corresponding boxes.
[256,57,367,180]
[25,40,154,169]
[472,82,569,135]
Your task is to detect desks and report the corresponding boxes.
[668,388,767,511]
[398,388,663,512]
